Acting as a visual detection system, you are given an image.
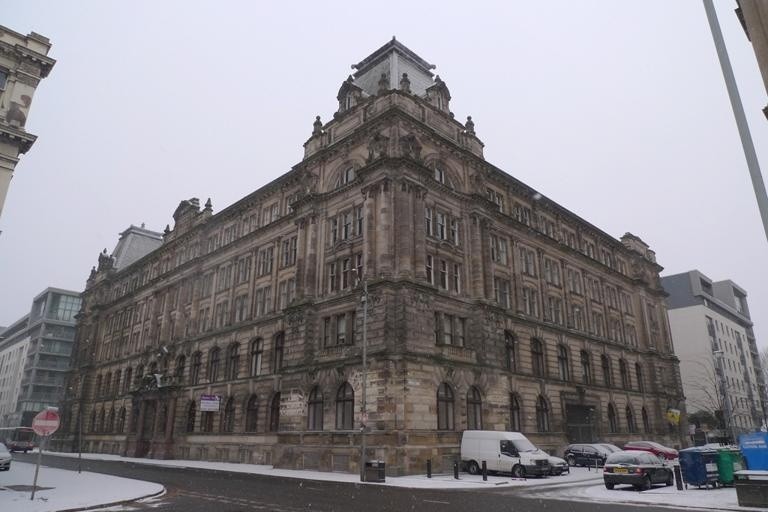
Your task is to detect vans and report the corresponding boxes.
[459,429,552,479]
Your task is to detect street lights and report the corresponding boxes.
[349,267,369,482]
[713,349,736,444]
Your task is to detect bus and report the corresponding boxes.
[0,426,36,454]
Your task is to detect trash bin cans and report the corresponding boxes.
[365,460,385,482]
[678,446,742,490]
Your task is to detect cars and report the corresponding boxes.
[600,444,622,453]
[602,448,673,492]
[540,449,569,477]
[622,440,678,462]
[563,443,612,467]
[0,442,12,471]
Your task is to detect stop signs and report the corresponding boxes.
[32,409,60,437]
